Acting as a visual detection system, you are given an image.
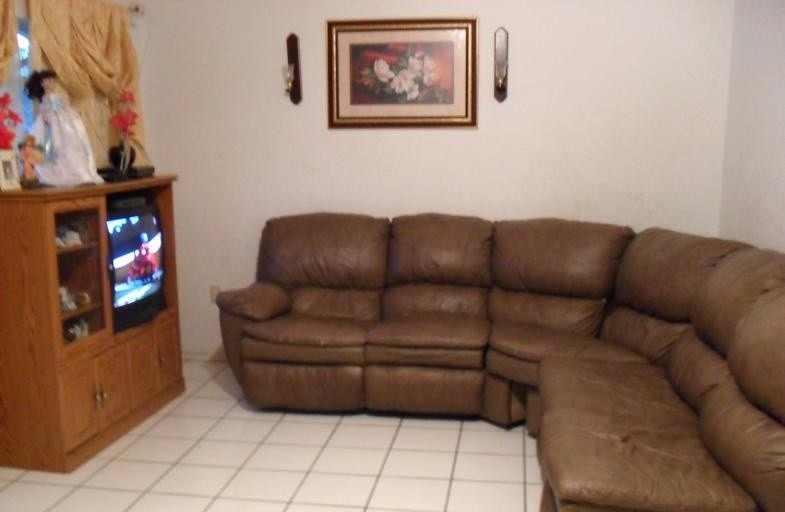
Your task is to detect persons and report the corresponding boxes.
[23,68,107,190]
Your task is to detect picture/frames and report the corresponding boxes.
[326,19,479,128]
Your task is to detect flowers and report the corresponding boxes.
[106,88,153,173]
[356,42,452,104]
[1,91,24,150]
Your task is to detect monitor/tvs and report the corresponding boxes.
[106,204,167,335]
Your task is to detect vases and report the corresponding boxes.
[108,140,137,171]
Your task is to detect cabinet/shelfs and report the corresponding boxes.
[0,171,186,474]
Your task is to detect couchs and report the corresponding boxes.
[214,210,492,412]
[479,219,785,512]
